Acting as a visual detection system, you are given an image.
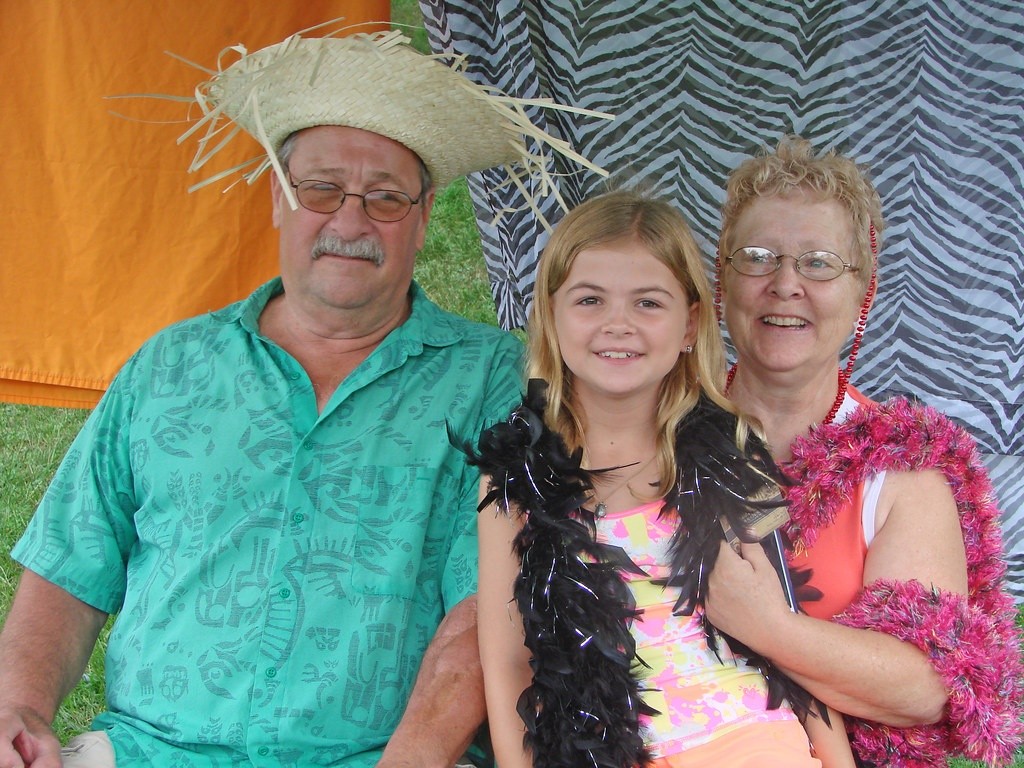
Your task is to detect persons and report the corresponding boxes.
[380,136,1024,766]
[445,183,856,767]
[0,16,617,768]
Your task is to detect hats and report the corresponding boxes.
[106,16,615,234]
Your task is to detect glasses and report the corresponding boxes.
[722,245,864,281]
[284,166,427,222]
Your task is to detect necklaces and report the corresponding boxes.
[712,211,878,455]
